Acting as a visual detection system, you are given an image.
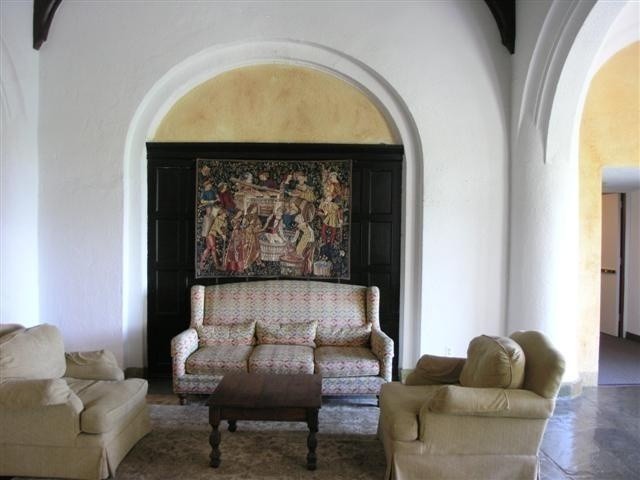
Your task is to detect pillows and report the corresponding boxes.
[0,324,67,381]
[255,319,318,348]
[196,319,257,348]
[315,322,373,347]
[458,334,526,388]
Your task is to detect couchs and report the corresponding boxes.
[0,323,151,480]
[170,280,395,406]
[378,329,567,480]
